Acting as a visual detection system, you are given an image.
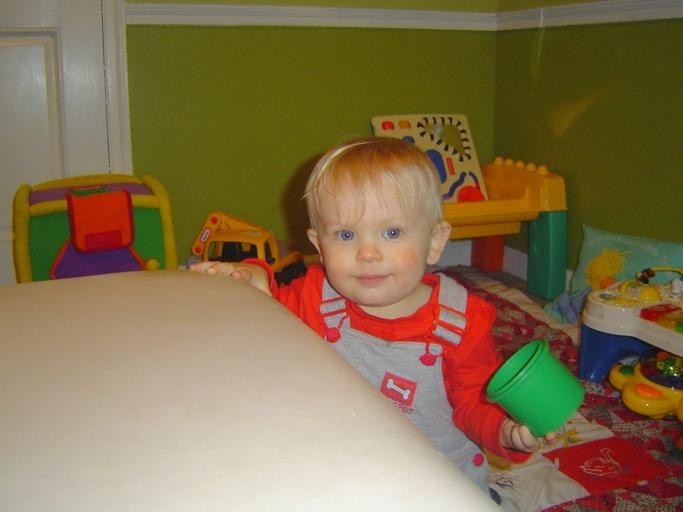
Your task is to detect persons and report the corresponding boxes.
[190,135,558,507]
